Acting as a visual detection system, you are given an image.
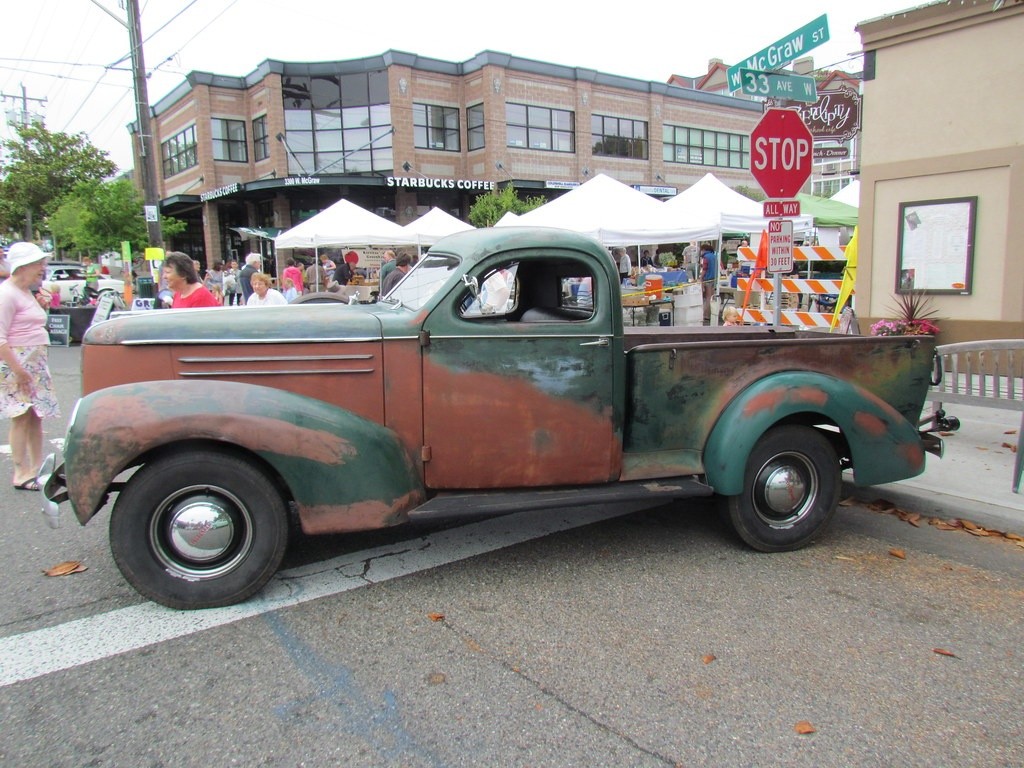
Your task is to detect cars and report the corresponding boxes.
[40,266,125,311]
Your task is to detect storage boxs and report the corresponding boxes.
[658,308,671,326]
[353,274,365,285]
[684,283,703,294]
[730,276,748,288]
[674,294,704,308]
[673,306,705,322]
[672,321,703,327]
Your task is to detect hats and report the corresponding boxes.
[312,258,316,263]
[7,242,52,275]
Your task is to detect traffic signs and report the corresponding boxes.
[739,68,819,105]
[726,13,829,92]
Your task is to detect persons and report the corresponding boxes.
[641,249,661,267]
[0,242,61,491]
[784,261,800,309]
[239,254,262,305]
[482,268,514,314]
[722,306,739,326]
[192,259,211,287]
[700,245,717,320]
[737,240,749,268]
[248,272,288,305]
[705,241,711,246]
[81,257,100,303]
[152,286,174,308]
[561,277,592,308]
[42,284,60,314]
[611,247,631,285]
[0,247,10,285]
[379,250,413,304]
[282,254,354,305]
[162,251,221,309]
[204,260,242,306]
[681,241,698,269]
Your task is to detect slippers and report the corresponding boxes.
[15,477,40,491]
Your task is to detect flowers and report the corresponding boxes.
[869,280,943,335]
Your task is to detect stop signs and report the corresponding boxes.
[748,107,814,200]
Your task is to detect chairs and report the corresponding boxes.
[520,307,577,322]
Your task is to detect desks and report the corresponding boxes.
[50,307,97,343]
[344,284,379,301]
[622,293,675,325]
[720,286,737,322]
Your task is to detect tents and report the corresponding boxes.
[492,172,860,244]
[274,198,421,246]
[403,206,477,247]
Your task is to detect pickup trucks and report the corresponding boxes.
[44,226,960,611]
